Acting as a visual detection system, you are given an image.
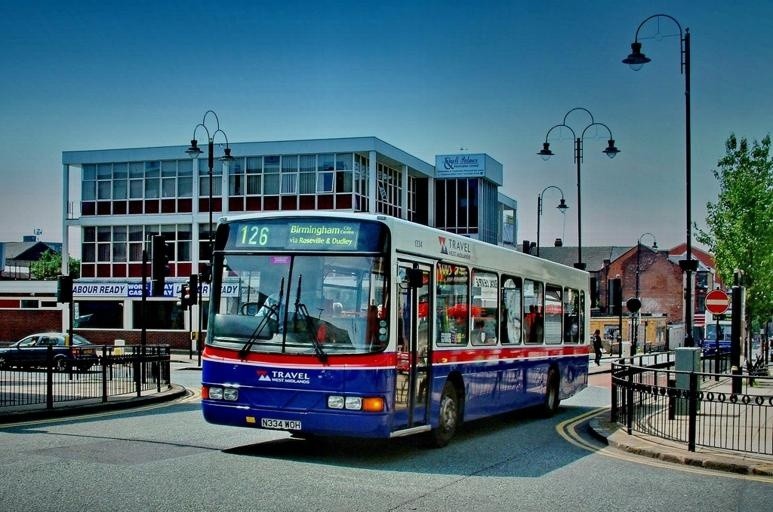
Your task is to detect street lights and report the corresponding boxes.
[620,11,698,349]
[536,106,623,269]
[184,110,238,270]
[634,232,657,349]
[601,256,610,313]
[534,186,569,257]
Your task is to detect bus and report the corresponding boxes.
[702,309,750,362]
[199,210,590,448]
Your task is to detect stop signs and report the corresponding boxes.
[704,290,728,315]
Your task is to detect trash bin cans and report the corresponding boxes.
[621,340,631,358]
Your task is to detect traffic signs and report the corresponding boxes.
[713,314,731,320]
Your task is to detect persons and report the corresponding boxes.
[255,279,306,335]
[376,303,386,318]
[592,329,604,366]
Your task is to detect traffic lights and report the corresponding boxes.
[176,284,186,310]
[185,274,198,304]
[151,235,169,298]
[56,276,71,302]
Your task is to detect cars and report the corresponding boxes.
[0,331,96,371]
[751,338,773,362]
